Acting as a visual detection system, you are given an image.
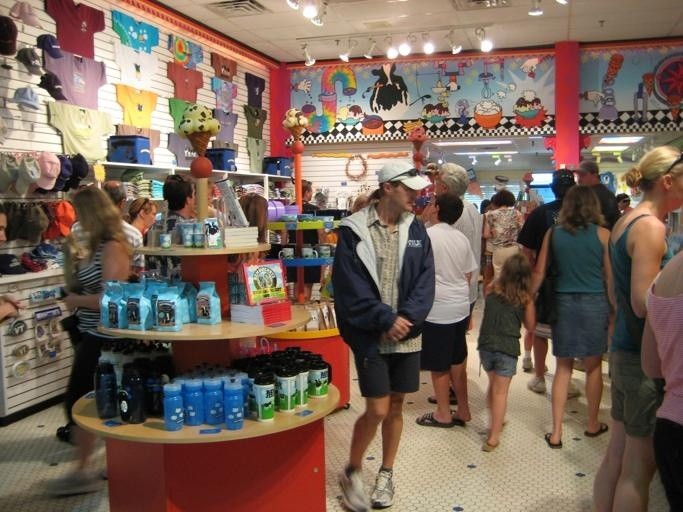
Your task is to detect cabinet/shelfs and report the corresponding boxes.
[93,160,293,283]
[265,222,351,412]
[667,207,683,236]
[0,147,74,418]
[71,103,341,511]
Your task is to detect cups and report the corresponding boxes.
[278,246,330,260]
[160,223,206,248]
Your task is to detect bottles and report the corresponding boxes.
[94,340,333,431]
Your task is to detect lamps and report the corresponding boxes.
[437,152,512,166]
[296,26,494,67]
[287,0,327,26]
[592,152,636,164]
[528,0,543,15]
[481,0,509,9]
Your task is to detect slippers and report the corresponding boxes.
[417,390,464,428]
[585,423,608,436]
[483,442,497,452]
[545,433,562,448]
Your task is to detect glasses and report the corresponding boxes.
[388,169,416,182]
[664,152,683,174]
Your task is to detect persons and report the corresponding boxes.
[640,250,683,512]
[0,203,27,324]
[592,145,683,512]
[332,160,432,511]
[56,185,136,444]
[413,160,630,452]
[70,176,318,276]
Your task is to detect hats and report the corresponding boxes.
[0,3,67,109]
[379,159,431,190]
[0,201,75,274]
[0,152,88,193]
[574,161,598,176]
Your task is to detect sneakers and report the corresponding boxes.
[340,467,393,512]
[57,424,78,445]
[523,358,579,399]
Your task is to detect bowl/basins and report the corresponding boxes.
[297,214,314,221]
[282,214,298,222]
